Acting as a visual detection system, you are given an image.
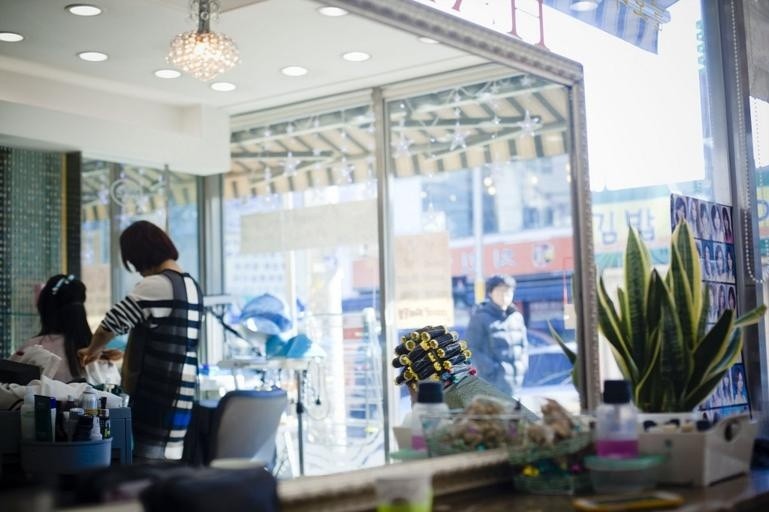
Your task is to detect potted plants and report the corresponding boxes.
[594,216,769,432]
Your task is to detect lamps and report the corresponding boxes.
[168,0,242,85]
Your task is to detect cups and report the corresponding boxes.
[375,469,432,511]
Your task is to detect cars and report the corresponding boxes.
[448,327,578,387]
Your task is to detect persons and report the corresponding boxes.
[77,220,204,459]
[672,194,752,421]
[392,324,539,423]
[7,273,121,386]
[466,274,528,397]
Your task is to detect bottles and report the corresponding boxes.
[408,381,451,450]
[594,380,639,458]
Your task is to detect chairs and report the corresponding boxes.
[191,389,285,473]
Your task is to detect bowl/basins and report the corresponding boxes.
[585,455,666,499]
